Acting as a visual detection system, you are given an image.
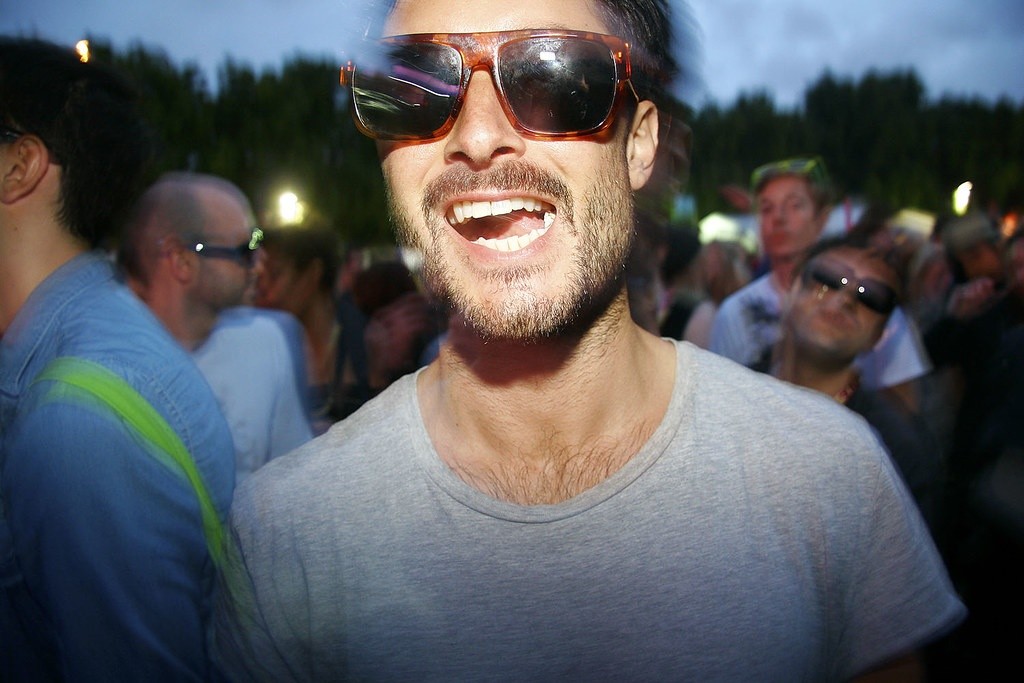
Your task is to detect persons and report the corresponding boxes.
[1,36,239,683]
[124,172,312,490]
[205,0,970,683]
[246,155,1024,563]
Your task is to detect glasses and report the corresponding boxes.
[802,259,898,315]
[179,243,259,267]
[750,157,815,190]
[335,29,640,142]
